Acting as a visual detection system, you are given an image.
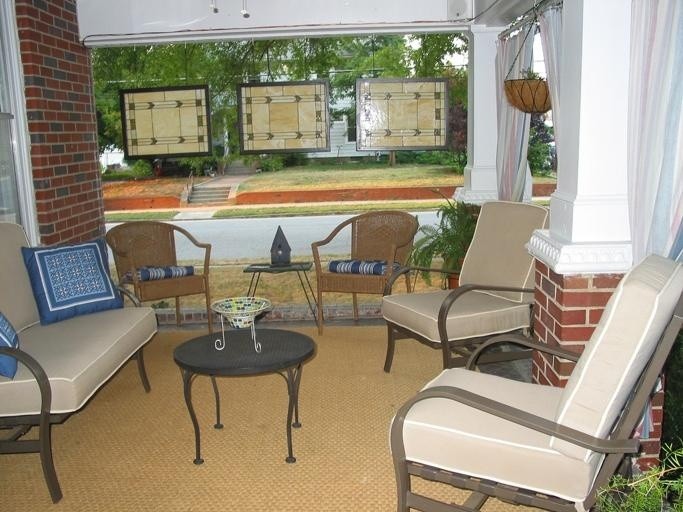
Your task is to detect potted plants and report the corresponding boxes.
[404,186,481,290]
[504,67,551,114]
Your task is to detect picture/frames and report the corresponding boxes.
[234,78,330,155]
[119,83,212,160]
[354,76,448,152]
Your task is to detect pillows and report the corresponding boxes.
[0,311,23,380]
[17,236,122,326]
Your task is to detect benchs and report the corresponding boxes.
[0,220,159,504]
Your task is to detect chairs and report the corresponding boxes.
[380,199,550,374]
[105,220,212,334]
[385,255,682,512]
[310,211,418,336]
